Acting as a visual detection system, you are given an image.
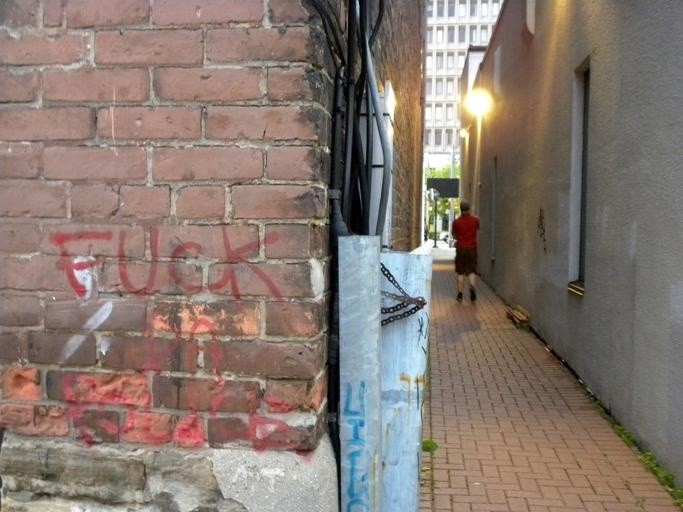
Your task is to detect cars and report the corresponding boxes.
[439,231,448,243]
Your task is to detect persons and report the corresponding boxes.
[452,201,480,302]
